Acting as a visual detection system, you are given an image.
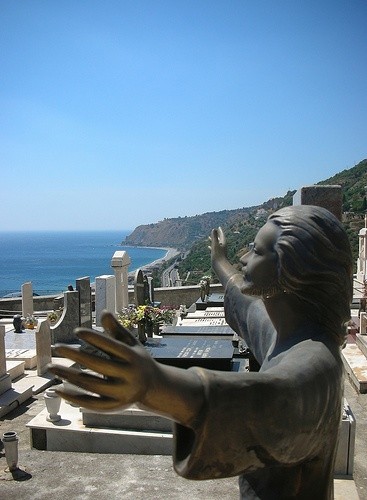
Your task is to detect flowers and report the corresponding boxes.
[49,313,57,322]
[118,309,136,331]
[199,280,207,288]
[203,276,212,287]
[24,313,36,323]
[134,305,151,323]
[149,308,161,323]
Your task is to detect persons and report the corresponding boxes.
[34,204,353,500]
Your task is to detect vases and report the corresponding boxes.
[200,287,206,301]
[171,313,178,326]
[27,323,34,329]
[1,431,21,472]
[146,323,154,338]
[205,285,210,299]
[13,315,24,333]
[51,323,54,327]
[44,388,62,422]
[137,323,148,345]
